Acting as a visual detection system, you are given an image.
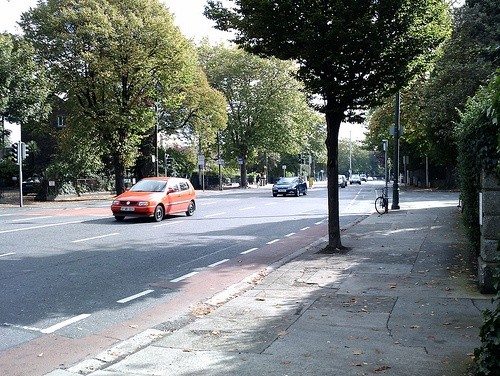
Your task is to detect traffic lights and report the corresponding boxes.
[21,142,30,161]
[11,142,19,165]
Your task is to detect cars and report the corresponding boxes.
[271,176,308,197]
[338,176,346,188]
[338,175,348,186]
[110,176,196,222]
[349,174,378,185]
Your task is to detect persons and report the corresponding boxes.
[309,176,315,189]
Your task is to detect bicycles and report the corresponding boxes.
[374,187,390,215]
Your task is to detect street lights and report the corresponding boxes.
[381,139,388,201]
